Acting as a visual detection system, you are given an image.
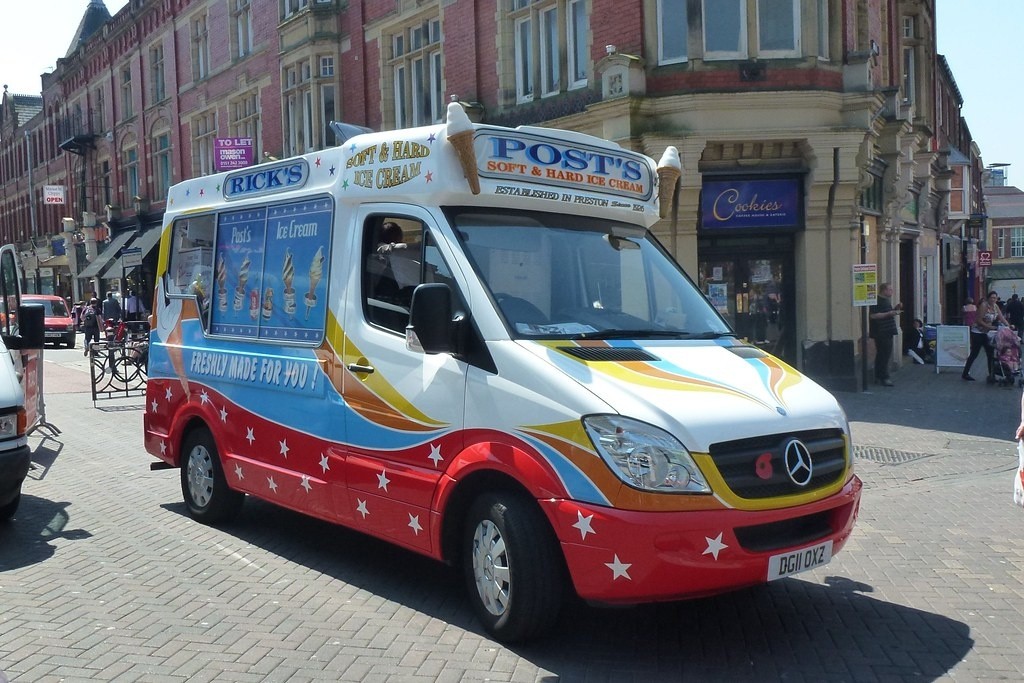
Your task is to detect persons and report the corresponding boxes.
[70,301,85,334]
[962,291,1015,381]
[962,296,977,353]
[1006,294,1024,345]
[383,222,404,244]
[128,290,146,341]
[1,310,19,337]
[869,283,903,386]
[977,296,1024,329]
[904,318,934,365]
[80,298,105,356]
[121,315,153,366]
[102,292,121,349]
[999,347,1020,374]
[86,292,102,313]
[732,271,784,344]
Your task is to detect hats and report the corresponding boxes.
[912,319,923,327]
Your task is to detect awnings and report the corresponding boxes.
[76,223,163,280]
[39,255,69,268]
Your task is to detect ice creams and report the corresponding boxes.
[218,252,227,317]
[657,146,681,219]
[446,102,482,194]
[156,274,191,402]
[304,245,323,320]
[234,251,252,317]
[282,246,295,320]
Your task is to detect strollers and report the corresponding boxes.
[986,326,1024,388]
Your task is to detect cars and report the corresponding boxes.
[9,294,77,348]
[0,243,46,521]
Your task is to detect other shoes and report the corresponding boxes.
[875,378,894,386]
[962,375,975,380]
[84,347,89,356]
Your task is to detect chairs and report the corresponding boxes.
[498,296,550,329]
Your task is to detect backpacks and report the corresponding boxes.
[84,306,96,327]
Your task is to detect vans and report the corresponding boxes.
[143,101,864,648]
